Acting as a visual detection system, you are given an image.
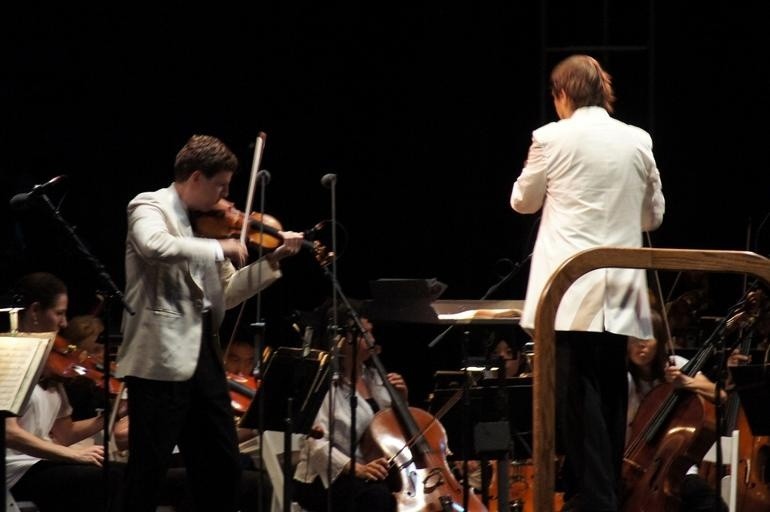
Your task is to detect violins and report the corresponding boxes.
[40,335,123,396]
[223,372,259,412]
[192,198,337,267]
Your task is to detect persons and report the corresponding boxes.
[0,269,131,512]
[511,54,667,511]
[291,300,408,512]
[221,323,259,441]
[623,270,770,512]
[491,340,519,378]
[113,132,306,511]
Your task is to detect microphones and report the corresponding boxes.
[10,173,68,209]
[320,172,337,185]
[257,170,271,185]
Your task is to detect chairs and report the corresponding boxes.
[258,429,306,512]
[700,430,739,512]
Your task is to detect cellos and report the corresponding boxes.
[359,344,485,512]
[617,287,764,509]
[487,444,566,510]
